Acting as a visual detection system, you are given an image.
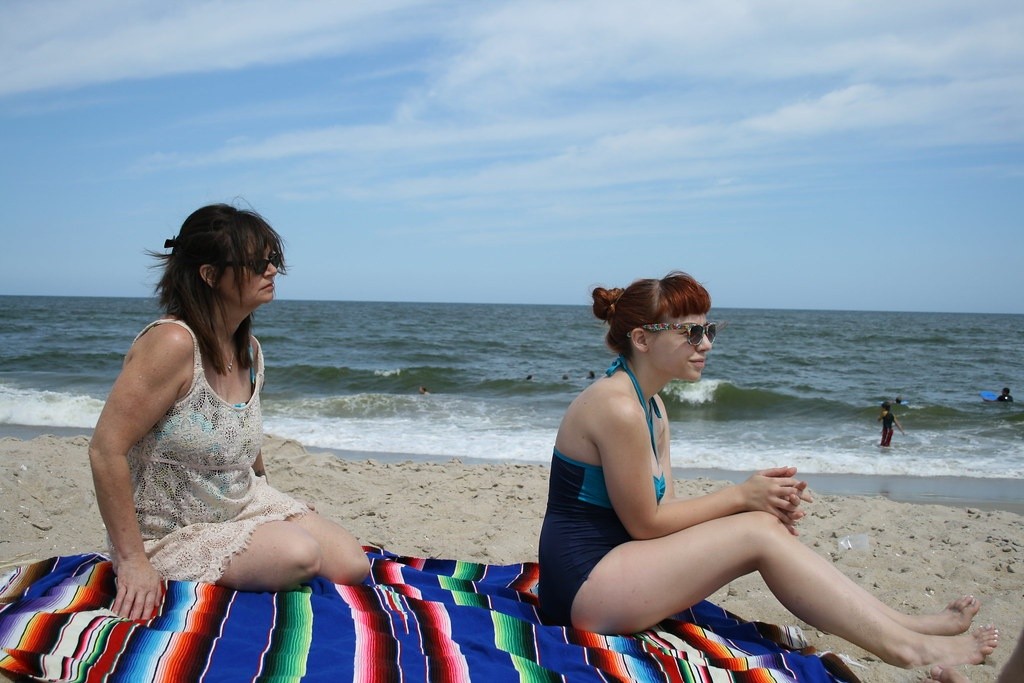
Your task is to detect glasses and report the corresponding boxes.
[194,253,279,275]
[627,322,717,347]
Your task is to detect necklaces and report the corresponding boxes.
[218,345,235,372]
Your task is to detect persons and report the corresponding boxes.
[86,203,370,621]
[419,386,430,395]
[537,271,997,671]
[879,402,906,448]
[983,387,1014,405]
